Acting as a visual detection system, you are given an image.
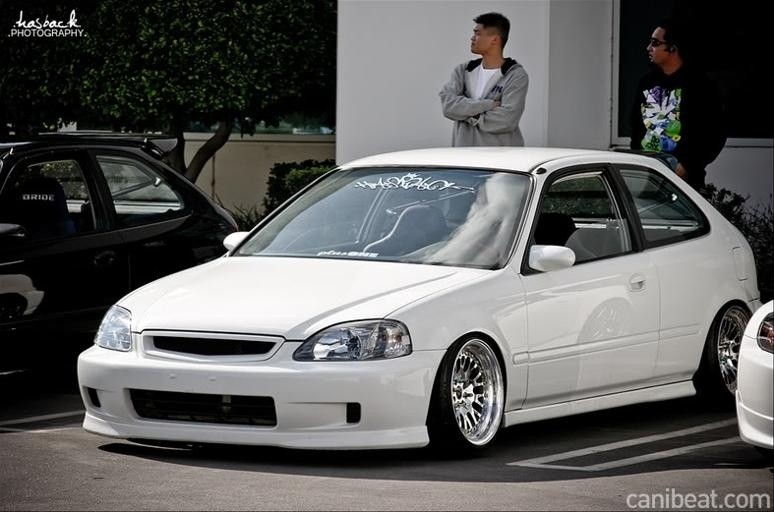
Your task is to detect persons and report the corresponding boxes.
[630,14,733,192]
[434,9,529,147]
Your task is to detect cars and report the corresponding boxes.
[0,132,774,458]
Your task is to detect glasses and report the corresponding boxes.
[649,36,668,48]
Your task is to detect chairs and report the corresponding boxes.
[362,204,453,256]
[534,211,577,246]
